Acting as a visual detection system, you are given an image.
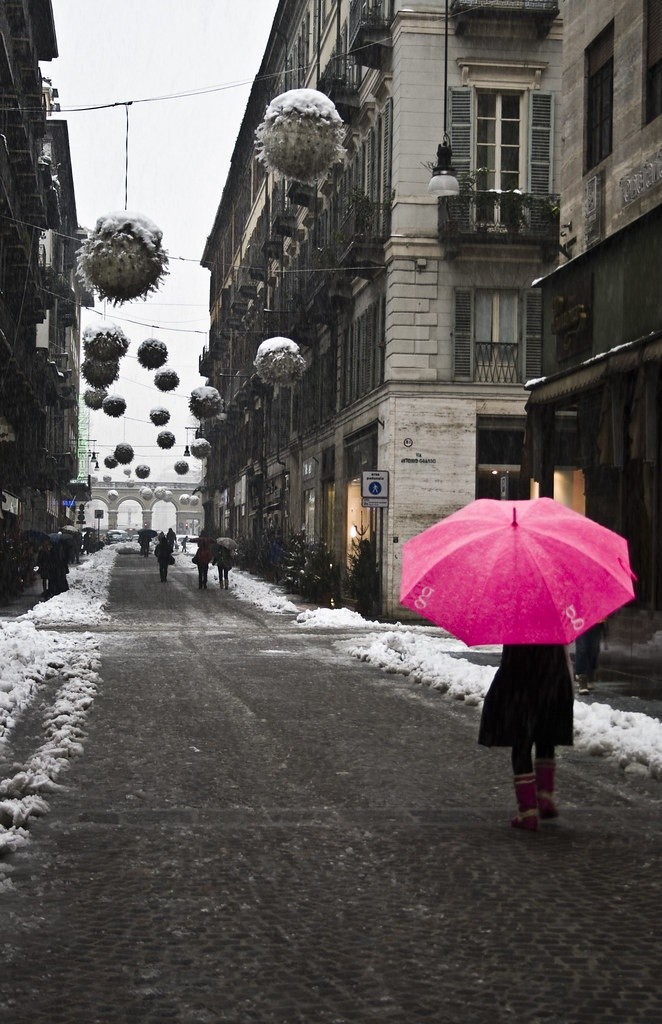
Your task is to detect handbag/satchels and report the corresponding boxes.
[168,556,175,565]
[223,559,232,571]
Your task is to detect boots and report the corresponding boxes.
[220,581,223,589]
[535,759,559,820]
[578,675,590,695]
[225,579,228,589]
[587,671,595,689]
[511,773,538,831]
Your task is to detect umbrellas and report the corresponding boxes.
[399,498,637,648]
[137,528,157,536]
[217,537,237,549]
[49,525,95,545]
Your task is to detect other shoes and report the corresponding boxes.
[204,586,207,589]
[199,586,202,589]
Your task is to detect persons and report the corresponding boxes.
[138,528,233,589]
[575,619,606,695]
[38,531,100,598]
[478,644,575,832]
